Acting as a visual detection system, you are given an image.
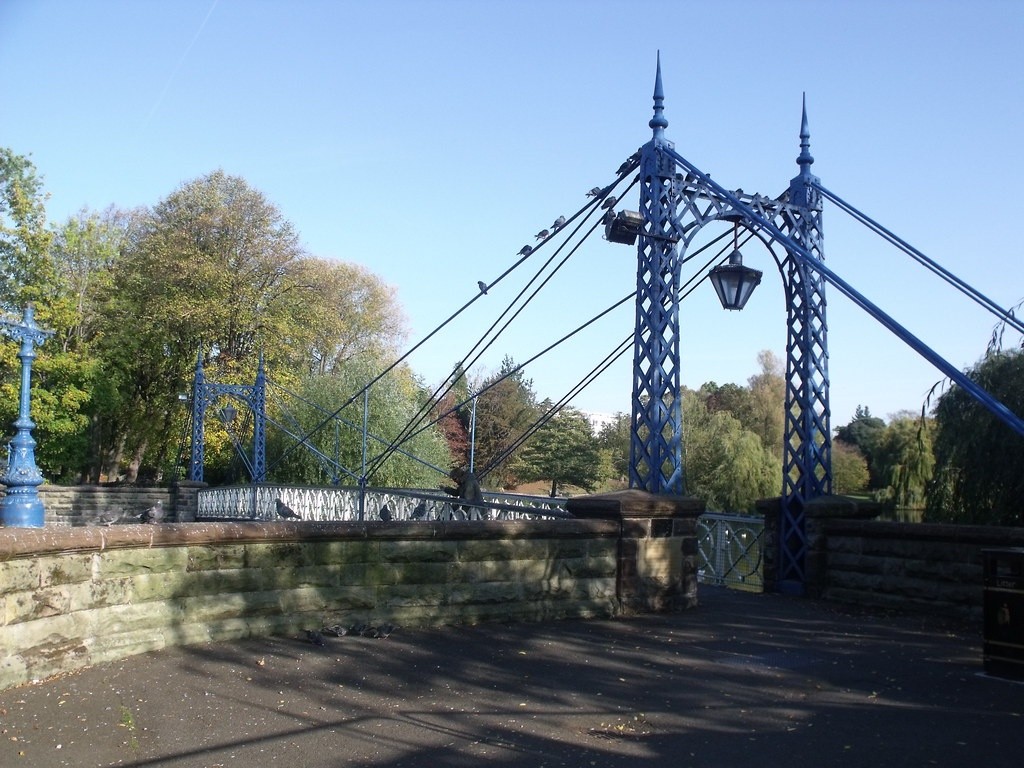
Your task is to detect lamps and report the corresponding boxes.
[708,220,763,312]
[221,397,238,422]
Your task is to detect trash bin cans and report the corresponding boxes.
[980,545,1024,681]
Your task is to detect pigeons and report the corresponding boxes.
[139,499,164,525]
[274,498,301,521]
[516,245,532,256]
[299,623,401,646]
[550,216,566,232]
[409,503,426,521]
[676,171,791,203]
[380,505,393,521]
[586,146,641,224]
[534,229,549,242]
[477,281,488,295]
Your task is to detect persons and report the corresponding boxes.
[443,467,488,521]
